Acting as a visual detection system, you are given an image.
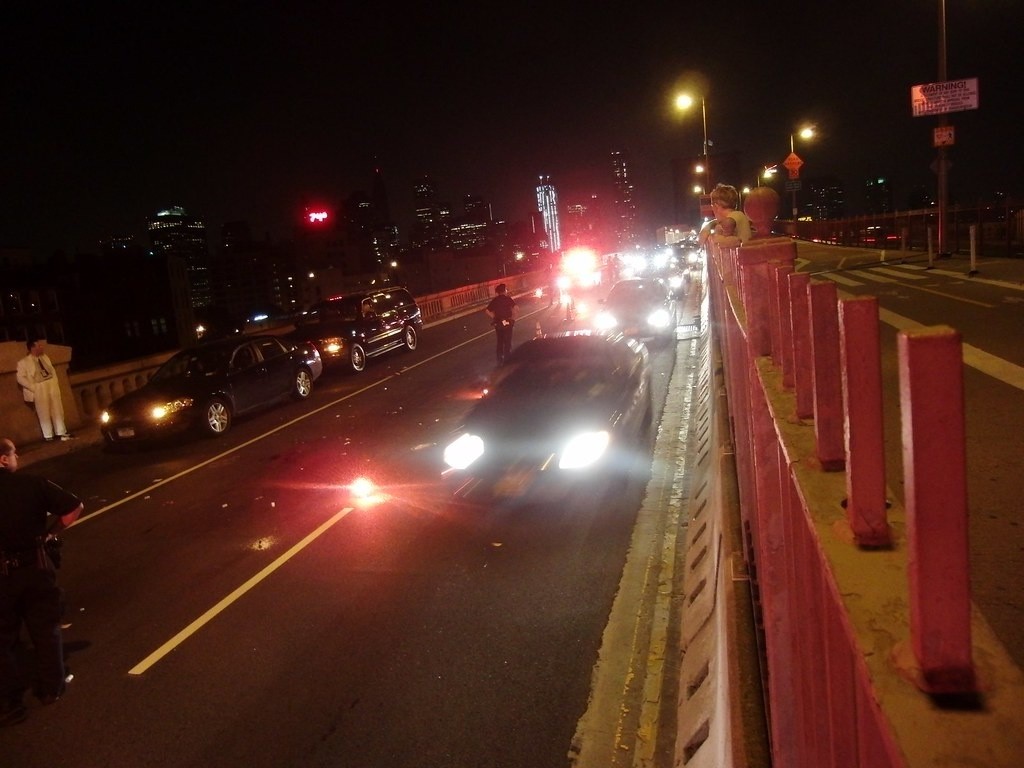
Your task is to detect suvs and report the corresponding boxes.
[283,285,424,372]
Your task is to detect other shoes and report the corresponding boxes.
[59,433,73,438]
[45,437,54,441]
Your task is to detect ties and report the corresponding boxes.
[38,358,49,376]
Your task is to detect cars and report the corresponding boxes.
[642,235,700,294]
[439,332,652,488]
[595,278,681,345]
[99,327,324,446]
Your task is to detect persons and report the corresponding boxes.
[697,183,753,245]
[0,436,84,726]
[16,339,72,441]
[485,284,519,368]
[606,254,621,283]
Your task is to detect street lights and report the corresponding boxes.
[673,91,710,194]
[789,128,816,235]
